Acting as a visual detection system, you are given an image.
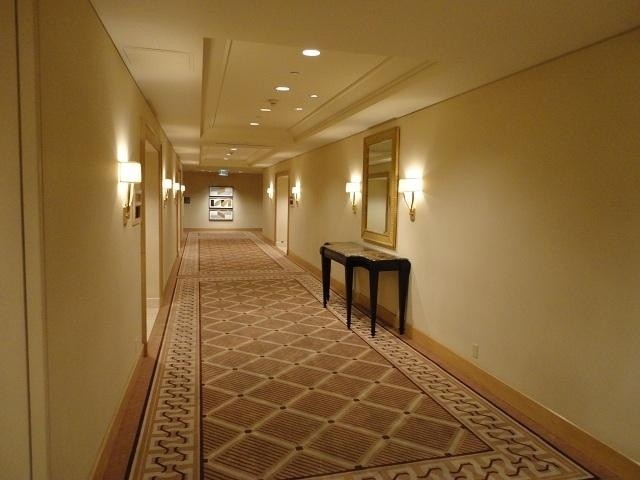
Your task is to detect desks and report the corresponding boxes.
[319,241,410,338]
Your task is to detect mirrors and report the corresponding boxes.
[360,126,402,249]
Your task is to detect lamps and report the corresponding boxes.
[162,176,172,203]
[112,145,146,228]
[397,161,425,221]
[344,169,362,215]
